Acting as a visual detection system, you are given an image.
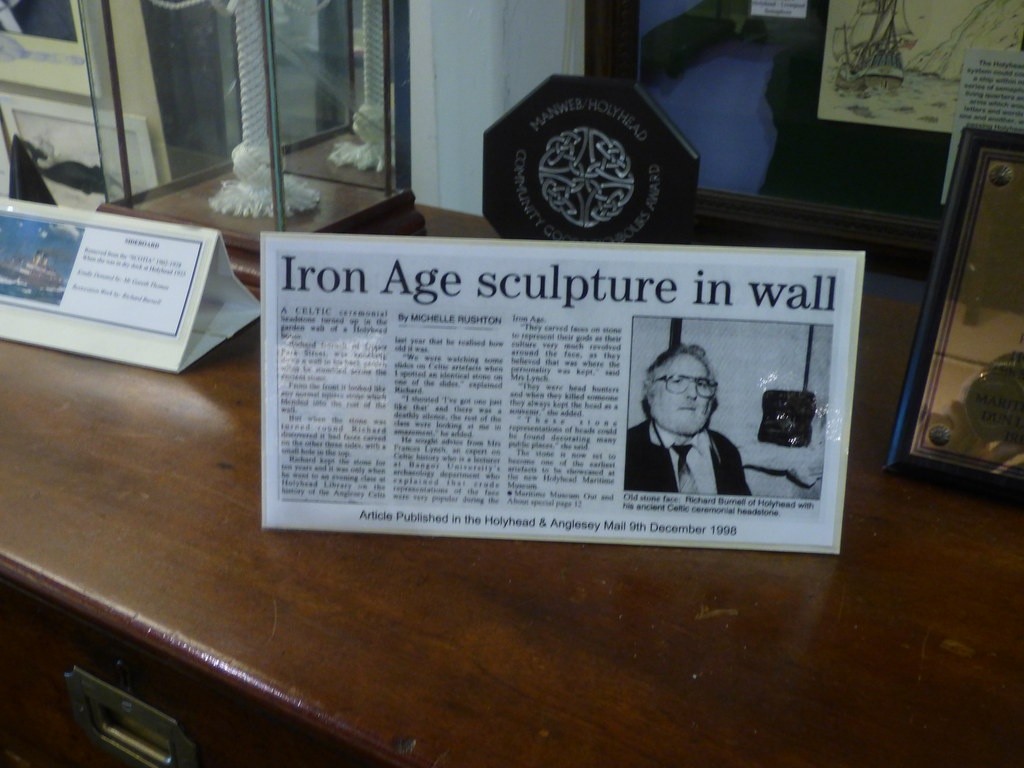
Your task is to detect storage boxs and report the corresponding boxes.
[78,0,428,303]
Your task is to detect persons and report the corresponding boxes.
[624,340,752,496]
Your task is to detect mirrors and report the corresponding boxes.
[584,0,1024,283]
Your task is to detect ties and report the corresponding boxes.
[671,444,697,493]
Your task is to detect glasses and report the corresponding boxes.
[651,374,718,398]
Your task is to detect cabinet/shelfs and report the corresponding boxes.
[0,203,1024,768]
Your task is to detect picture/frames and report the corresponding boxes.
[882,128,1024,511]
